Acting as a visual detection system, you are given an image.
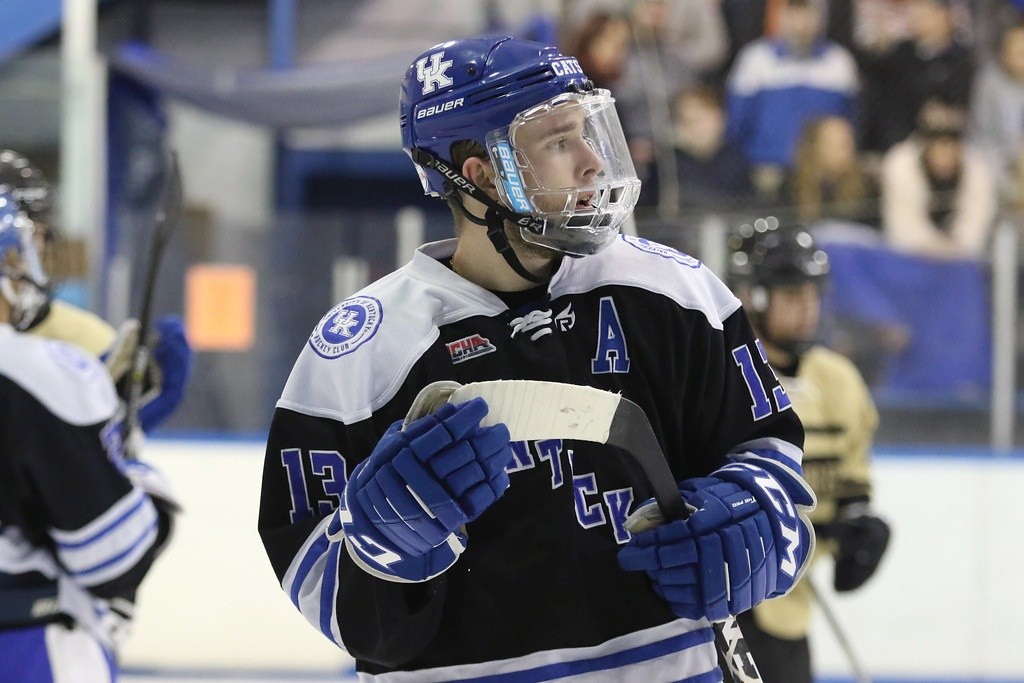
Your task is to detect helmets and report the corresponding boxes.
[401,35,641,258]
[0,149,52,192]
[726,219,828,286]
[0,189,29,279]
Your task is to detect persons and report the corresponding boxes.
[0,186,185,683]
[0,148,193,438]
[496,0,1024,252]
[723,216,890,683]
[257,32,817,683]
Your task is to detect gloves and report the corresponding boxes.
[822,515,890,591]
[617,452,816,623]
[325,396,512,584]
[99,319,192,438]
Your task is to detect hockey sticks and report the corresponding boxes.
[800,574,865,680]
[106,145,185,623]
[445,378,768,683]
